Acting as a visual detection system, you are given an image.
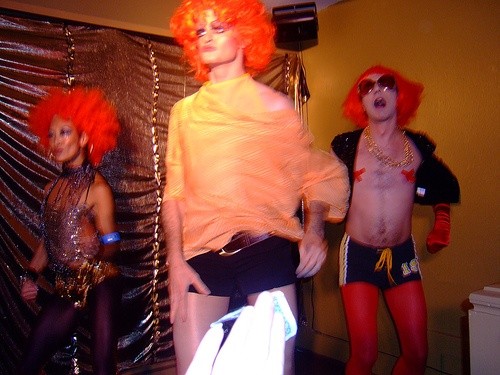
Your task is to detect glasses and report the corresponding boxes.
[358,74,396,95]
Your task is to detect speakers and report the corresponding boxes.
[272,2,318,51]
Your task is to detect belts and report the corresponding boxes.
[216,231,275,256]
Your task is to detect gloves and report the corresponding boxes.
[426,203,451,253]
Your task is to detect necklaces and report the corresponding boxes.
[359,124,415,169]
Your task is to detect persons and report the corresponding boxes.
[327,65,461,374]
[163,1,351,374]
[18,87,116,375]
